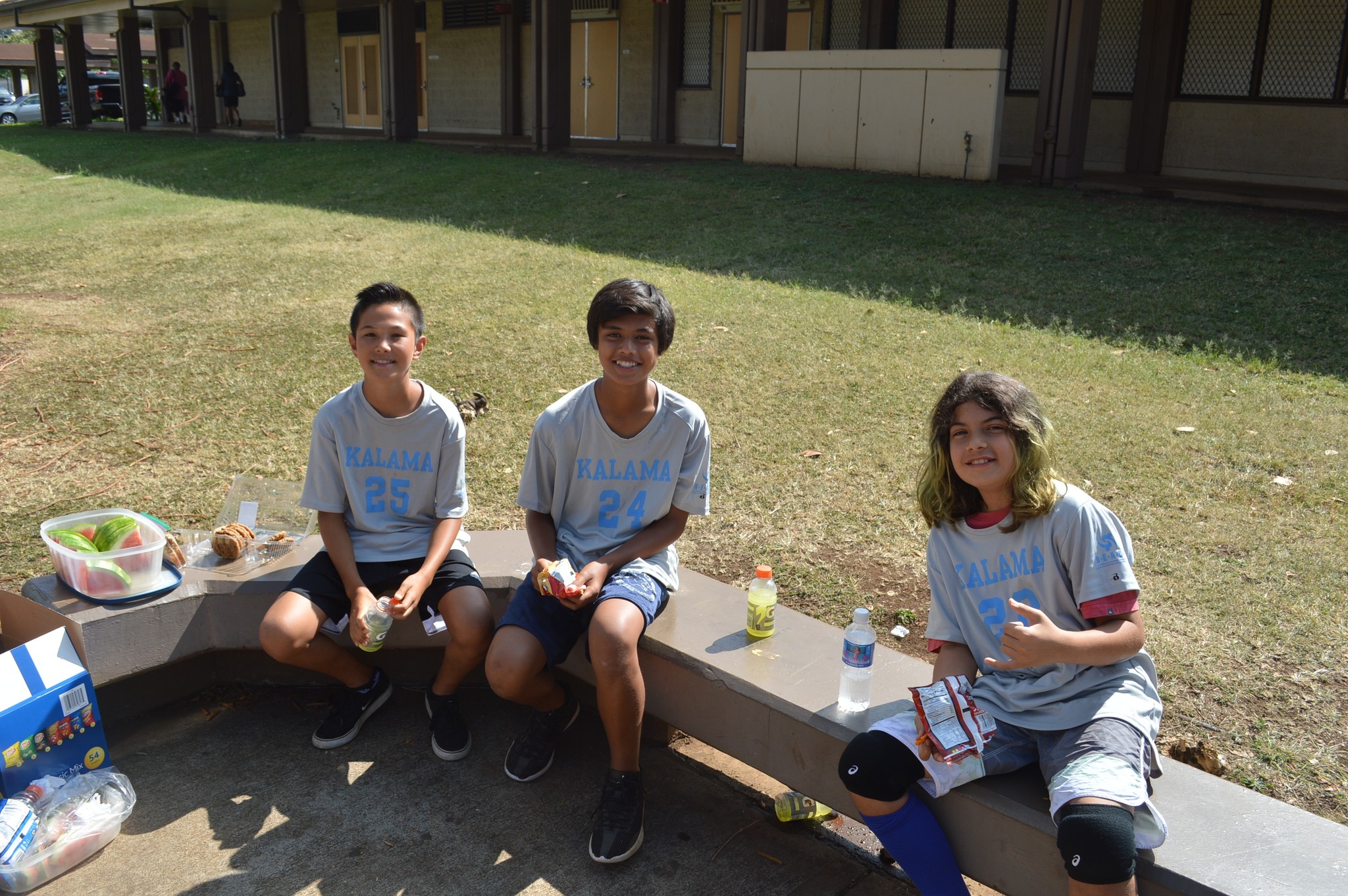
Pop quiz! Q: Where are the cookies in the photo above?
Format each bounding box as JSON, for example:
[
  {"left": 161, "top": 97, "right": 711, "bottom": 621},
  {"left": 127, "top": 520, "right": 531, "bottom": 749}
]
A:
[
  {"left": 258, "top": 530, "right": 296, "bottom": 554},
  {"left": 210, "top": 524, "right": 255, "bottom": 559}
]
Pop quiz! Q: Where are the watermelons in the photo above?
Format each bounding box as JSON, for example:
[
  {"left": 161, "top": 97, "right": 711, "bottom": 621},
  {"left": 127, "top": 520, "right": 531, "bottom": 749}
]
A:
[{"left": 45, "top": 514, "right": 150, "bottom": 598}]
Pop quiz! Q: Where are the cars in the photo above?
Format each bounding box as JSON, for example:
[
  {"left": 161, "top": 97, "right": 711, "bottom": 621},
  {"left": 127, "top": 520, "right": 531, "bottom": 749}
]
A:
[
  {"left": 0, "top": 93, "right": 43, "bottom": 124},
  {"left": 0, "top": 86, "right": 16, "bottom": 106}
]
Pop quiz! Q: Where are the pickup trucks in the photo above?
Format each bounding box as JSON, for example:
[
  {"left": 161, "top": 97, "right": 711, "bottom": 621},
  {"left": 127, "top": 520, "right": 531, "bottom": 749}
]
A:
[{"left": 58, "top": 71, "right": 151, "bottom": 123}]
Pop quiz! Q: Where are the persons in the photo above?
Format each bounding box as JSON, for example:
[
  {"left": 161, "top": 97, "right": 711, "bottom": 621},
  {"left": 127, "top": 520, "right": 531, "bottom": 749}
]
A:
[
  {"left": 163, "top": 61, "right": 187, "bottom": 125},
  {"left": 218, "top": 62, "right": 244, "bottom": 127},
  {"left": 839, "top": 370, "right": 1168, "bottom": 896},
  {"left": 257, "top": 282, "right": 486, "bottom": 762},
  {"left": 485, "top": 277, "right": 711, "bottom": 863}
]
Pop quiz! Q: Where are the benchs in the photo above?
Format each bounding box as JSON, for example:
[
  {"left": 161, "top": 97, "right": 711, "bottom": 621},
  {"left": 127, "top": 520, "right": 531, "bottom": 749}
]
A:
[{"left": 0, "top": 520, "right": 1348, "bottom": 896}]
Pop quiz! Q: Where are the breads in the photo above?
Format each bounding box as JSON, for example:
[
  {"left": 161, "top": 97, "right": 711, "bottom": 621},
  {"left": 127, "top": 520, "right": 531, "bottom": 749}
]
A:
[{"left": 164, "top": 533, "right": 186, "bottom": 568}]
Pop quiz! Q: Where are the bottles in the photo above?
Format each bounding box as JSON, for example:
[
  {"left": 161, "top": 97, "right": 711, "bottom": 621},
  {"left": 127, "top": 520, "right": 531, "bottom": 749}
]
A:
[
  {"left": 775, "top": 791, "right": 832, "bottom": 821},
  {"left": 359, "top": 596, "right": 395, "bottom": 652},
  {"left": 747, "top": 565, "right": 777, "bottom": 638},
  {"left": 10, "top": 785, "right": 43, "bottom": 816},
  {"left": 838, "top": 608, "right": 877, "bottom": 712}
]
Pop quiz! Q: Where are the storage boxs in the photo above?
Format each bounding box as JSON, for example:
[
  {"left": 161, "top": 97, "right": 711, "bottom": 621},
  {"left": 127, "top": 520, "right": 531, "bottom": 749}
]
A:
[
  {"left": 0, "top": 769, "right": 124, "bottom": 894},
  {"left": 0, "top": 625, "right": 116, "bottom": 805},
  {"left": 37, "top": 503, "right": 184, "bottom": 606}
]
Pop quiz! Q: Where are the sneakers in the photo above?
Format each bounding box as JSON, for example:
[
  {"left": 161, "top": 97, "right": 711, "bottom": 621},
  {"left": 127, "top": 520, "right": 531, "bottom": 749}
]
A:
[
  {"left": 504, "top": 691, "right": 579, "bottom": 781},
  {"left": 588, "top": 769, "right": 646, "bottom": 862},
  {"left": 424, "top": 687, "right": 473, "bottom": 761},
  {"left": 312, "top": 668, "right": 392, "bottom": 749}
]
